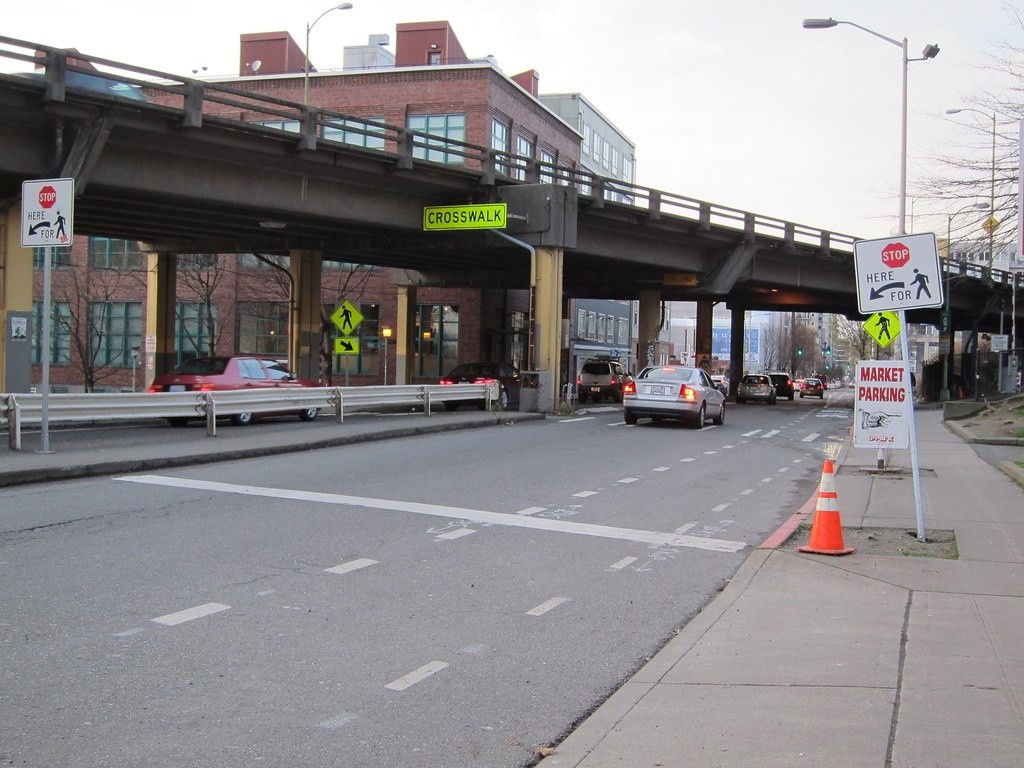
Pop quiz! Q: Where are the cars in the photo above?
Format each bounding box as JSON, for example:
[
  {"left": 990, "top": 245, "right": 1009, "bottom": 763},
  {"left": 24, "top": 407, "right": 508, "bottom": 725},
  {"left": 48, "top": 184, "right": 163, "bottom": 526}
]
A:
[
  {"left": 623, "top": 365, "right": 725, "bottom": 428},
  {"left": 149, "top": 356, "right": 324, "bottom": 425},
  {"left": 440, "top": 362, "right": 522, "bottom": 407},
  {"left": 709, "top": 373, "right": 731, "bottom": 399},
  {"left": 736, "top": 374, "right": 778, "bottom": 404},
  {"left": 792, "top": 378, "right": 805, "bottom": 391},
  {"left": 800, "top": 378, "right": 824, "bottom": 399}
]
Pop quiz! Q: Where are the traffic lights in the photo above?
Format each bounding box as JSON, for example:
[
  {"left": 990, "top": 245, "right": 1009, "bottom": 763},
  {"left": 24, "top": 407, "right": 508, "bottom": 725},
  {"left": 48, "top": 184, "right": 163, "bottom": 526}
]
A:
[
  {"left": 796, "top": 347, "right": 804, "bottom": 355},
  {"left": 825, "top": 364, "right": 830, "bottom": 369},
  {"left": 821, "top": 346, "right": 831, "bottom": 356}
]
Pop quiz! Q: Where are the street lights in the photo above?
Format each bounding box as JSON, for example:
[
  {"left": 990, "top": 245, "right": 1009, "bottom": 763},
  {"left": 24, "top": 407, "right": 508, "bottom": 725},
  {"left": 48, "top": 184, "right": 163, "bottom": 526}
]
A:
[
  {"left": 304, "top": 3, "right": 353, "bottom": 107},
  {"left": 946, "top": 108, "right": 996, "bottom": 279},
  {"left": 910, "top": 190, "right": 944, "bottom": 235},
  {"left": 937, "top": 203, "right": 991, "bottom": 401},
  {"left": 802, "top": 16, "right": 940, "bottom": 236}
]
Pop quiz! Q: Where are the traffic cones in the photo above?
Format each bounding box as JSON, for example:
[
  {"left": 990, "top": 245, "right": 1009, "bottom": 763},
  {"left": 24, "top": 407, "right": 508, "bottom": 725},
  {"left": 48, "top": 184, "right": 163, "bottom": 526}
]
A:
[{"left": 796, "top": 460, "right": 855, "bottom": 554}]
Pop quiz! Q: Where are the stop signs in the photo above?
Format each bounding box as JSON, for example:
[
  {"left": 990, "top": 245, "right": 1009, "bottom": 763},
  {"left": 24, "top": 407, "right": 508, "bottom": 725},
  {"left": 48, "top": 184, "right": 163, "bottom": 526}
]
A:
[
  {"left": 38, "top": 185, "right": 58, "bottom": 208},
  {"left": 881, "top": 242, "right": 911, "bottom": 269}
]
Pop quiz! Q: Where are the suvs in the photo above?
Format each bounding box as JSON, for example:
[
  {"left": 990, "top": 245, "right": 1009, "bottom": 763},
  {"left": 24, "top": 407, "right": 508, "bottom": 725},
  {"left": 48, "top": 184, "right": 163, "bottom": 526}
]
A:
[
  {"left": 769, "top": 373, "right": 796, "bottom": 400},
  {"left": 578, "top": 359, "right": 632, "bottom": 403}
]
